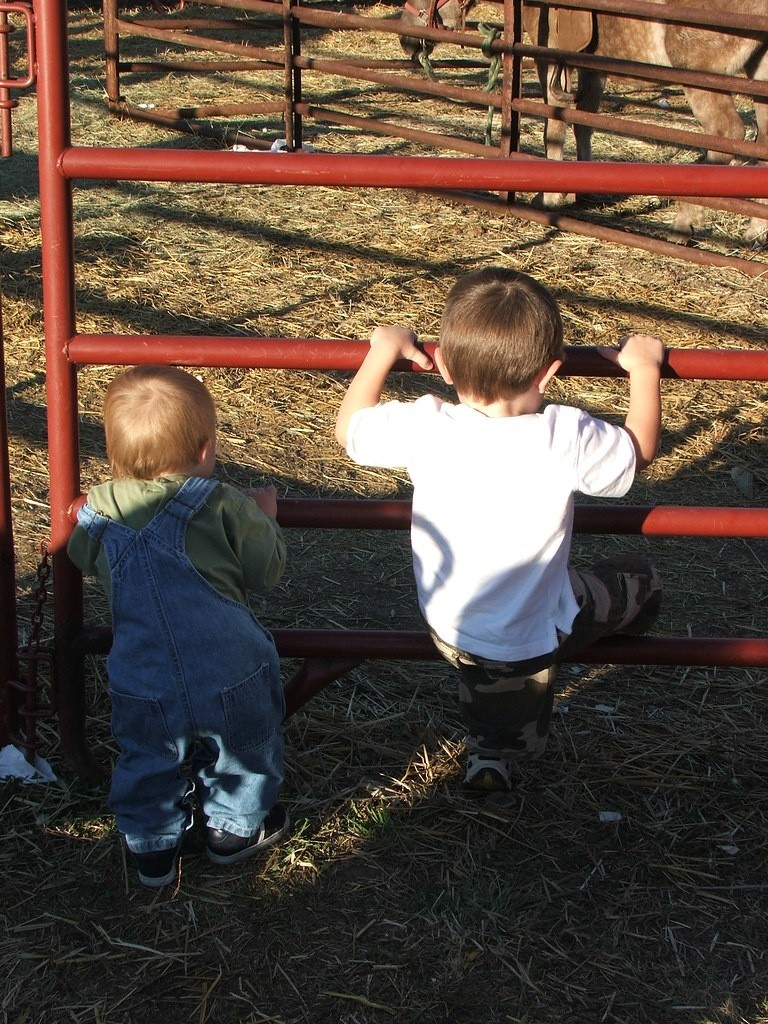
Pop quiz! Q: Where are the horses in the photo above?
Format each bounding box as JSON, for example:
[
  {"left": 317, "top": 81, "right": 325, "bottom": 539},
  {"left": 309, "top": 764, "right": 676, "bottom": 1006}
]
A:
[{"left": 398, "top": 0, "right": 768, "bottom": 247}]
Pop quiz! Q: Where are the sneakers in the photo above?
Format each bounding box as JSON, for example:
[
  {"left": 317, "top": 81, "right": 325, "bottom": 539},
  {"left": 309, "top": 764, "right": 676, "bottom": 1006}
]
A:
[
  {"left": 206, "top": 805, "right": 289, "bottom": 863},
  {"left": 136, "top": 845, "right": 181, "bottom": 887}
]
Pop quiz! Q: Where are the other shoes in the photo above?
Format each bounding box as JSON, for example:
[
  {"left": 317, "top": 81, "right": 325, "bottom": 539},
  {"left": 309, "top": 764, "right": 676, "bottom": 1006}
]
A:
[{"left": 463, "top": 756, "right": 514, "bottom": 793}]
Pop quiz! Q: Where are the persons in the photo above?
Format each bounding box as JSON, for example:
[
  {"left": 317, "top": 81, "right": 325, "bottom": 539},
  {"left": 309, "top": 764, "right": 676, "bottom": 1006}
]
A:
[
  {"left": 336, "top": 269, "right": 663, "bottom": 793},
  {"left": 66, "top": 365, "right": 290, "bottom": 888}
]
